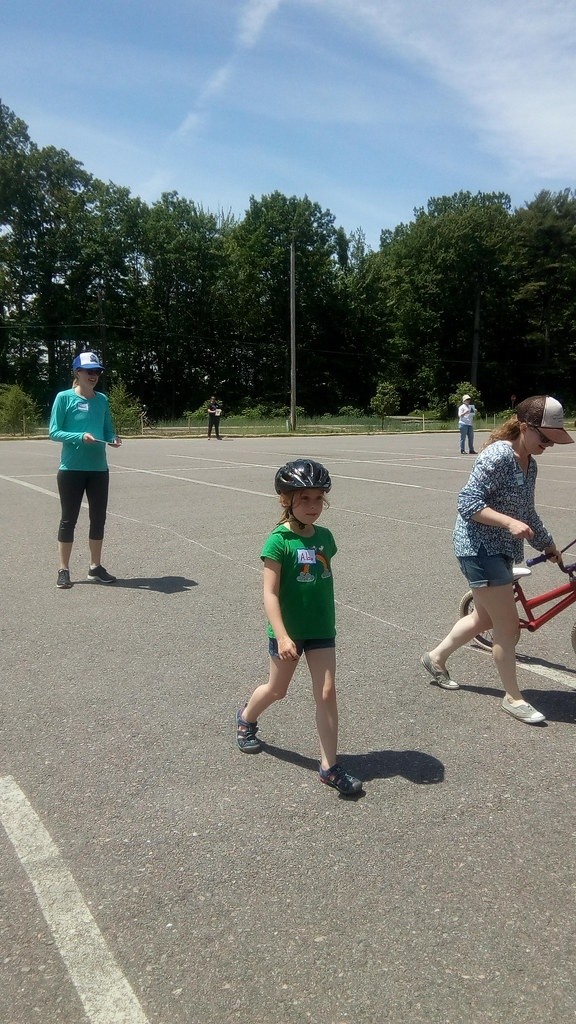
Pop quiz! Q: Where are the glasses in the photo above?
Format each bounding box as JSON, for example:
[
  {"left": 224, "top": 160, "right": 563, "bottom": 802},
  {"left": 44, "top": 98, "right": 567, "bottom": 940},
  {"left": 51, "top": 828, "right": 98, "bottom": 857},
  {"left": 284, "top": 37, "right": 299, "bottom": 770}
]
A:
[
  {"left": 78, "top": 368, "right": 103, "bottom": 376},
  {"left": 535, "top": 426, "right": 549, "bottom": 443}
]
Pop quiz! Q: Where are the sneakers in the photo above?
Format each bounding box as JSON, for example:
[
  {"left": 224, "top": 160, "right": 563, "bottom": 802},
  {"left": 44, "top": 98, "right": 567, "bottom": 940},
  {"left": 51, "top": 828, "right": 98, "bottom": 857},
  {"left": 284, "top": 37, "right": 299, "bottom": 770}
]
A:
[
  {"left": 501, "top": 697, "right": 545, "bottom": 723},
  {"left": 87, "top": 564, "right": 116, "bottom": 582},
  {"left": 56, "top": 569, "right": 71, "bottom": 588},
  {"left": 421, "top": 652, "right": 460, "bottom": 690}
]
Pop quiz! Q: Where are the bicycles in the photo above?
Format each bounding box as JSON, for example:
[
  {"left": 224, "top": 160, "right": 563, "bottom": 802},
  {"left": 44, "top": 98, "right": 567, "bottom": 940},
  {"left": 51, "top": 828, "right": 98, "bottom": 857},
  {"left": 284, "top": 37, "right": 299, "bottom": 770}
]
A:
[{"left": 458, "top": 539, "right": 576, "bottom": 655}]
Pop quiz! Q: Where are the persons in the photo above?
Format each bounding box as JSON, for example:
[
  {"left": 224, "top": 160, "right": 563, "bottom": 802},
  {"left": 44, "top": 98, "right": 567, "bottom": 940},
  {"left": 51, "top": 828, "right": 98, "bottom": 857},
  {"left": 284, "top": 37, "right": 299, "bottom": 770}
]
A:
[
  {"left": 235, "top": 458, "right": 363, "bottom": 795},
  {"left": 207, "top": 396, "right": 222, "bottom": 440},
  {"left": 456, "top": 395, "right": 478, "bottom": 455},
  {"left": 48, "top": 351, "right": 122, "bottom": 588},
  {"left": 418, "top": 395, "right": 575, "bottom": 724}
]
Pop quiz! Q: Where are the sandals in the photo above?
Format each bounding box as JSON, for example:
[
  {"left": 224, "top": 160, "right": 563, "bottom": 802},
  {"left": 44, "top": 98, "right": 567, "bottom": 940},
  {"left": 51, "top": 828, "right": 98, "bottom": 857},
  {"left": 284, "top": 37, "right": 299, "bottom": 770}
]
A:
[
  {"left": 235, "top": 703, "right": 261, "bottom": 754},
  {"left": 317, "top": 758, "right": 362, "bottom": 793}
]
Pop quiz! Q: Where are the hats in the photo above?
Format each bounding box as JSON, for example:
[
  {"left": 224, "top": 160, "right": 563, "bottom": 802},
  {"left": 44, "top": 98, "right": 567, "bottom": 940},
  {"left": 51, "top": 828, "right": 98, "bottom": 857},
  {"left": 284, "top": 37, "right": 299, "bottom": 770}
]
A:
[
  {"left": 462, "top": 394, "right": 471, "bottom": 403},
  {"left": 517, "top": 395, "right": 575, "bottom": 444},
  {"left": 72, "top": 352, "right": 105, "bottom": 372}
]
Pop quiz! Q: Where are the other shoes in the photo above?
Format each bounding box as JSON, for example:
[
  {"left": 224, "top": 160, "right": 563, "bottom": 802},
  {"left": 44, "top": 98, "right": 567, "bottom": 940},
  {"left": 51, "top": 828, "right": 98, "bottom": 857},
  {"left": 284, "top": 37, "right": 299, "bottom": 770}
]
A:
[
  {"left": 218, "top": 437, "right": 222, "bottom": 440},
  {"left": 208, "top": 437, "right": 210, "bottom": 440},
  {"left": 461, "top": 450, "right": 467, "bottom": 454},
  {"left": 469, "top": 450, "right": 478, "bottom": 454}
]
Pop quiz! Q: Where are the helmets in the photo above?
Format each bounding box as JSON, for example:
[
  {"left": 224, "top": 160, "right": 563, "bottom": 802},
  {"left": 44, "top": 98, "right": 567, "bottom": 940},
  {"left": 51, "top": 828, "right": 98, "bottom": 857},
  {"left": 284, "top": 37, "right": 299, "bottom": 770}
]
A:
[{"left": 274, "top": 458, "right": 331, "bottom": 495}]
